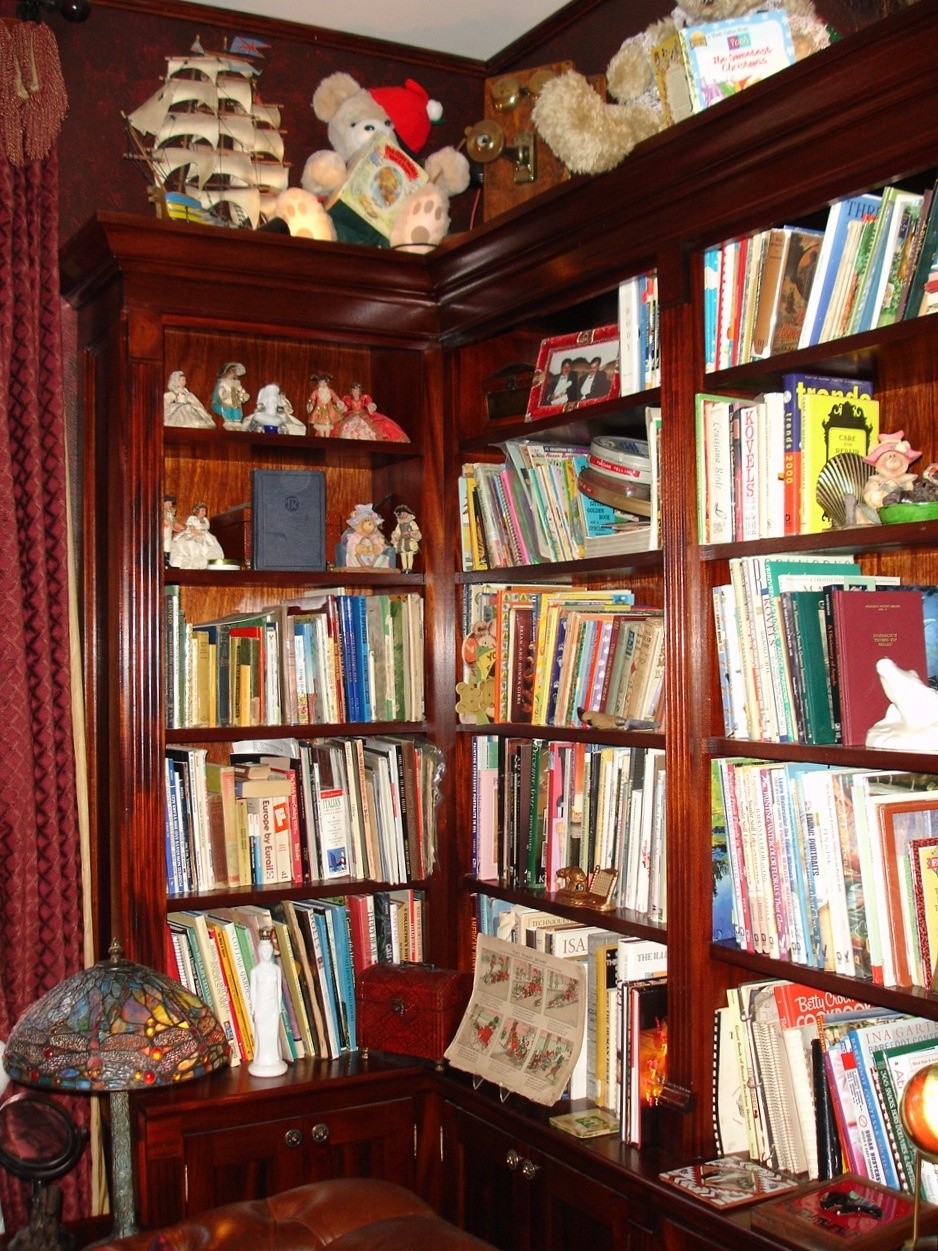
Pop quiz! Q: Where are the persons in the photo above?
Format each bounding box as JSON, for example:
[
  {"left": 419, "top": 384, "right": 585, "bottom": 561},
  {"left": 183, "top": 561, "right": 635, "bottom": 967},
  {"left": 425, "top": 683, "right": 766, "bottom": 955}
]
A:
[
  {"left": 306, "top": 369, "right": 345, "bottom": 437},
  {"left": 212, "top": 362, "right": 251, "bottom": 432},
  {"left": 389, "top": 504, "right": 423, "bottom": 574},
  {"left": 543, "top": 356, "right": 608, "bottom": 406},
  {"left": 330, "top": 381, "right": 411, "bottom": 444},
  {"left": 164, "top": 368, "right": 215, "bottom": 428},
  {"left": 241, "top": 381, "right": 308, "bottom": 437},
  {"left": 247, "top": 927, "right": 288, "bottom": 1078},
  {"left": 162, "top": 493, "right": 224, "bottom": 568}
]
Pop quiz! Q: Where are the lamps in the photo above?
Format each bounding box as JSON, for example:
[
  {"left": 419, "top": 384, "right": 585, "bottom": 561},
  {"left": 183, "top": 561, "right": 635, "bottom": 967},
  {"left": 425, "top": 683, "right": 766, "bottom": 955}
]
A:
[{"left": 4, "top": 938, "right": 231, "bottom": 1239}]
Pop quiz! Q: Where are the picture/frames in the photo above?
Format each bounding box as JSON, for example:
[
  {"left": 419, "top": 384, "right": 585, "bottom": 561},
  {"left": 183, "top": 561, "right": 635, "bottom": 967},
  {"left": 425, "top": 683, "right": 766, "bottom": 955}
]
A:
[{"left": 525, "top": 323, "right": 620, "bottom": 423}]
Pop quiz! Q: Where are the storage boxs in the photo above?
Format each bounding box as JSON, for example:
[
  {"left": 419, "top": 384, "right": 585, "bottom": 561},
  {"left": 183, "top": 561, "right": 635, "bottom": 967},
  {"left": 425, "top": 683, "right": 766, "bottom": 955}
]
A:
[{"left": 751, "top": 1173, "right": 938, "bottom": 1251}]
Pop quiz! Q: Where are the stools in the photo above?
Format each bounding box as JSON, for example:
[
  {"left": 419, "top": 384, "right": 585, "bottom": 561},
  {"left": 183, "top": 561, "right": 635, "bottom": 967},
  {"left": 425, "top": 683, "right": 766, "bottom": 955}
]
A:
[{"left": 84, "top": 1176, "right": 503, "bottom": 1251}]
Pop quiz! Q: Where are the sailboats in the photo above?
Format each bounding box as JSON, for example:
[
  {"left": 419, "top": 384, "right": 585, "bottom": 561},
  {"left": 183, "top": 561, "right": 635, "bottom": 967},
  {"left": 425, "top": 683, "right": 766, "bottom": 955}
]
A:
[{"left": 118, "top": 34, "right": 292, "bottom": 232}]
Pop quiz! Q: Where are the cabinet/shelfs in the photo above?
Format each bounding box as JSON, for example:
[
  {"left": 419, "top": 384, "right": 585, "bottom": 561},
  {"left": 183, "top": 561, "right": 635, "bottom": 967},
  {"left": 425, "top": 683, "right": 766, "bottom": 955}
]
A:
[{"left": 58, "top": 0, "right": 938, "bottom": 1251}]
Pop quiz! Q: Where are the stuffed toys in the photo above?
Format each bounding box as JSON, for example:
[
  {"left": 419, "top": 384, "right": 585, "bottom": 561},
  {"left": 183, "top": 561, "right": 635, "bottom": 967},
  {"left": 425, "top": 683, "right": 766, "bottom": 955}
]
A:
[
  {"left": 273, "top": 72, "right": 472, "bottom": 255},
  {"left": 530, "top": 0, "right": 831, "bottom": 176}
]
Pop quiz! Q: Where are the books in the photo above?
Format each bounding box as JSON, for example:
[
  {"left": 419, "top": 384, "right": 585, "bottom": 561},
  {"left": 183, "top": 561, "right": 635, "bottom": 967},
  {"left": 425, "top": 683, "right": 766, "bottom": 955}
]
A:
[
  {"left": 469, "top": 890, "right": 674, "bottom": 1151},
  {"left": 713, "top": 557, "right": 928, "bottom": 746},
  {"left": 694, "top": 373, "right": 879, "bottom": 544},
  {"left": 712, "top": 980, "right": 938, "bottom": 1203},
  {"left": 460, "top": 406, "right": 664, "bottom": 573},
  {"left": 616, "top": 266, "right": 663, "bottom": 398},
  {"left": 704, "top": 180, "right": 938, "bottom": 373},
  {"left": 164, "top": 735, "right": 443, "bottom": 895},
  {"left": 250, "top": 468, "right": 328, "bottom": 572},
  {"left": 460, "top": 582, "right": 667, "bottom": 730},
  {"left": 470, "top": 734, "right": 669, "bottom": 924},
  {"left": 710, "top": 758, "right": 938, "bottom": 990},
  {"left": 163, "top": 583, "right": 427, "bottom": 727},
  {"left": 165, "top": 887, "right": 425, "bottom": 1068}
]
